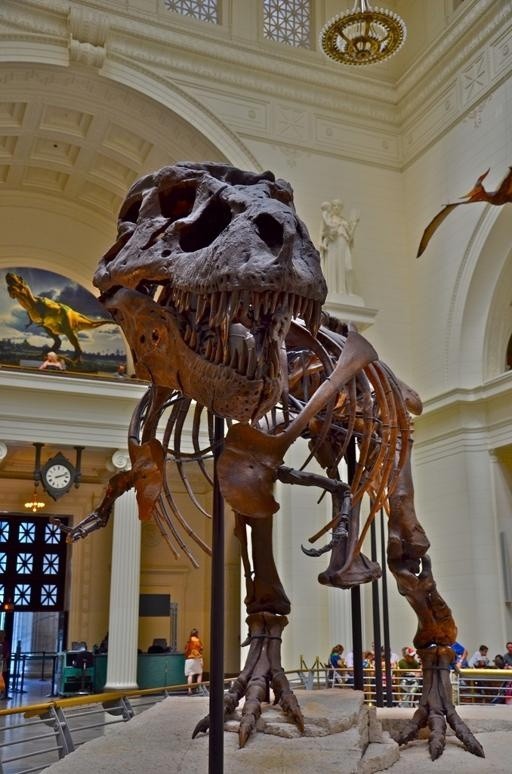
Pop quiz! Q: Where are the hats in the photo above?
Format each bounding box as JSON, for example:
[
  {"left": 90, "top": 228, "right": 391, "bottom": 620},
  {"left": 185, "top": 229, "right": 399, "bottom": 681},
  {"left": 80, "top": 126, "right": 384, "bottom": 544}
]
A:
[{"left": 402, "top": 647, "right": 416, "bottom": 656}]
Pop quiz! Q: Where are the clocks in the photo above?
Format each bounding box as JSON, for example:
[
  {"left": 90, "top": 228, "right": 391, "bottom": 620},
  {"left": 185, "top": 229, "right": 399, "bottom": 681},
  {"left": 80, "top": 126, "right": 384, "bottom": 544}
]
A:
[{"left": 32, "top": 442, "right": 86, "bottom": 502}]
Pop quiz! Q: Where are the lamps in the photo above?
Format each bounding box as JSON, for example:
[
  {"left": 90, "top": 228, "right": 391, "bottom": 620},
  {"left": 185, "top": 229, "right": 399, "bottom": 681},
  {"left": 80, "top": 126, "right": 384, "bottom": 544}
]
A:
[
  {"left": 318, "top": 0, "right": 408, "bottom": 68},
  {"left": 24, "top": 487, "right": 45, "bottom": 513}
]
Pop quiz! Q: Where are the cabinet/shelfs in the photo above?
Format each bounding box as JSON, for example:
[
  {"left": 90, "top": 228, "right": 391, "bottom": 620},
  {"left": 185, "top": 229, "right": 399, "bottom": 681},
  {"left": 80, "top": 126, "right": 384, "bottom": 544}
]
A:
[{"left": 59, "top": 651, "right": 95, "bottom": 697}]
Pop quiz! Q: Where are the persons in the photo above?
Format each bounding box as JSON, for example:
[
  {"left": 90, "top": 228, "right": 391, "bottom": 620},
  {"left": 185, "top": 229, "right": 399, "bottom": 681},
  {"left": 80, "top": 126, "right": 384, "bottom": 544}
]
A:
[
  {"left": 113, "top": 365, "right": 129, "bottom": 378},
  {"left": 318, "top": 198, "right": 361, "bottom": 297},
  {"left": 40, "top": 352, "right": 66, "bottom": 369},
  {"left": 328, "top": 644, "right": 420, "bottom": 706},
  {"left": 184, "top": 628, "right": 205, "bottom": 694},
  {"left": 448, "top": 641, "right": 512, "bottom": 704}
]
